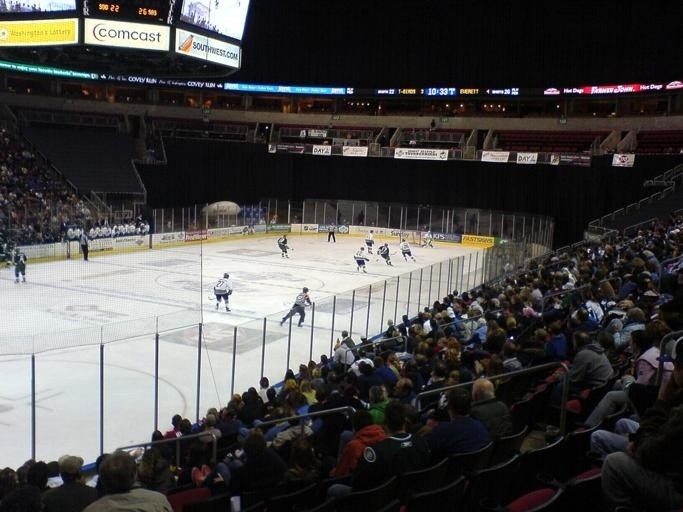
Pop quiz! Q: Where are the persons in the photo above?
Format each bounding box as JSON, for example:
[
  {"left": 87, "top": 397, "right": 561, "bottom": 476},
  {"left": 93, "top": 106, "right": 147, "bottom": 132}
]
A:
[
  {"left": 0, "top": 287, "right": 683, "bottom": 512},
  {"left": 480, "top": 214, "right": 682, "bottom": 288},
  {"left": 214, "top": 273, "right": 232, "bottom": 311},
  {"left": 377, "top": 244, "right": 393, "bottom": 267},
  {"left": 365, "top": 229, "right": 374, "bottom": 254},
  {"left": 1, "top": 101, "right": 149, "bottom": 264},
  {"left": 353, "top": 248, "right": 369, "bottom": 273},
  {"left": 276, "top": 233, "right": 293, "bottom": 259},
  {"left": 399, "top": 239, "right": 415, "bottom": 262},
  {"left": 164, "top": 212, "right": 429, "bottom": 235},
  {"left": 428, "top": 238, "right": 433, "bottom": 249},
  {"left": 326, "top": 118, "right": 436, "bottom": 147},
  {"left": 180, "top": 0, "right": 241, "bottom": 35},
  {"left": 0, "top": 0, "right": 49, "bottom": 13},
  {"left": 13, "top": 247, "right": 26, "bottom": 282},
  {"left": 327, "top": 222, "right": 337, "bottom": 243},
  {"left": 422, "top": 237, "right": 427, "bottom": 248}
]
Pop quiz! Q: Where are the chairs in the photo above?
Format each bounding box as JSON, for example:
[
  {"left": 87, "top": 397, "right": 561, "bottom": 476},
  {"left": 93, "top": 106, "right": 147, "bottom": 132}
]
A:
[{"left": 169, "top": 210, "right": 682, "bottom": 511}]
